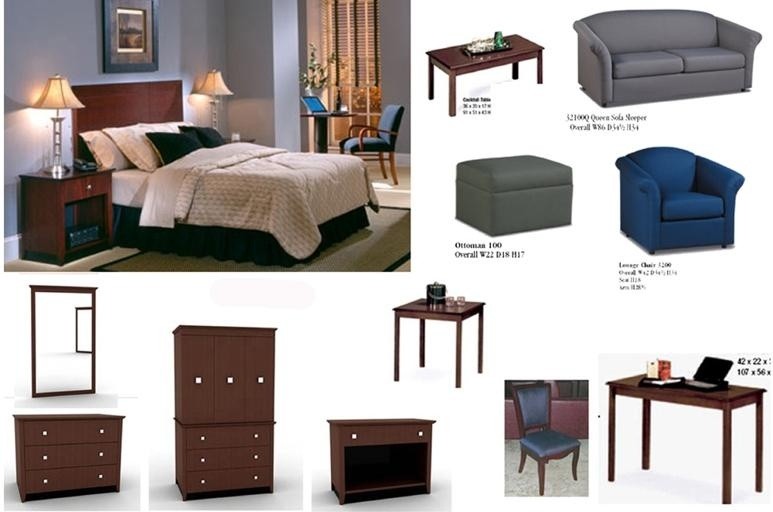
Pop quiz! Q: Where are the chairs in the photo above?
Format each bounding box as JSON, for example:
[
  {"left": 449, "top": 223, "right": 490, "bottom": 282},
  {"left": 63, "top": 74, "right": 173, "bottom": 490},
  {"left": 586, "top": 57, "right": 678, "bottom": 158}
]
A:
[
  {"left": 508, "top": 382, "right": 581, "bottom": 497},
  {"left": 338, "top": 104, "right": 404, "bottom": 186}
]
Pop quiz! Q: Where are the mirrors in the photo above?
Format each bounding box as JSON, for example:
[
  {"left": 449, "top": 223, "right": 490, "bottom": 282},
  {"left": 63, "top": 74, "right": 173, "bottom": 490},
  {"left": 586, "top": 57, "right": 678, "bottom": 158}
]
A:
[
  {"left": 28, "top": 284, "right": 98, "bottom": 399},
  {"left": 74, "top": 306, "right": 92, "bottom": 353}
]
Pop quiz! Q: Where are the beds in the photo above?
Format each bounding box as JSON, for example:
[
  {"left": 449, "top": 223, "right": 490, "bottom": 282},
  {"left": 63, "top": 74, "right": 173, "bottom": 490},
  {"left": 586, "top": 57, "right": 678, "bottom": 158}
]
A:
[{"left": 71, "top": 80, "right": 380, "bottom": 268}]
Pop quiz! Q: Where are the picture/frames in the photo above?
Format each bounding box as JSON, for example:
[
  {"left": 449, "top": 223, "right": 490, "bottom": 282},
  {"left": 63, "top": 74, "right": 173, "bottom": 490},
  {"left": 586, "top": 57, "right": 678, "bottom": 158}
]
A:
[{"left": 102, "top": 0, "right": 159, "bottom": 74}]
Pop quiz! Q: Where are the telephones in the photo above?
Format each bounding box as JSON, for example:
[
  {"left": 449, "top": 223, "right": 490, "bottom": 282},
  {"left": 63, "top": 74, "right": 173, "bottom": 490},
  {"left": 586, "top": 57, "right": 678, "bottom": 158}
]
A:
[{"left": 72, "top": 158, "right": 98, "bottom": 173}]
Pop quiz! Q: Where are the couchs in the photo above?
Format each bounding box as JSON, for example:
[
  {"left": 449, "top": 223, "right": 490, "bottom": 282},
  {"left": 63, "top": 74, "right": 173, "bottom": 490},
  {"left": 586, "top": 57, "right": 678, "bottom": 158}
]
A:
[
  {"left": 455, "top": 156, "right": 573, "bottom": 238},
  {"left": 571, "top": 8, "right": 761, "bottom": 108},
  {"left": 615, "top": 147, "right": 746, "bottom": 255}
]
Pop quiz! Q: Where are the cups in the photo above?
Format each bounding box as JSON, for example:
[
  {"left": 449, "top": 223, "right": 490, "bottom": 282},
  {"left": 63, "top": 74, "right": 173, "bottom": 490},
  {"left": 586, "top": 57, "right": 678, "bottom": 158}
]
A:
[
  {"left": 456, "top": 295, "right": 465, "bottom": 307},
  {"left": 340, "top": 103, "right": 348, "bottom": 112},
  {"left": 230, "top": 130, "right": 240, "bottom": 142},
  {"left": 445, "top": 297, "right": 453, "bottom": 307}
]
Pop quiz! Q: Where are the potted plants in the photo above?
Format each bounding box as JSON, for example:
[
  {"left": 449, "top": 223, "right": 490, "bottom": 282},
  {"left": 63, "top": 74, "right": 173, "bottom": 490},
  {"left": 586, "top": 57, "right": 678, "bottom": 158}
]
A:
[{"left": 299, "top": 43, "right": 350, "bottom": 115}]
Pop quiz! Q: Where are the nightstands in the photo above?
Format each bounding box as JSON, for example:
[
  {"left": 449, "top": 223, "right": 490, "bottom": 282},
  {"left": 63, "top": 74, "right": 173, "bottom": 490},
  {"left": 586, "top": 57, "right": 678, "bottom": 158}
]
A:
[
  {"left": 218, "top": 136, "right": 256, "bottom": 144},
  {"left": 17, "top": 167, "right": 116, "bottom": 267}
]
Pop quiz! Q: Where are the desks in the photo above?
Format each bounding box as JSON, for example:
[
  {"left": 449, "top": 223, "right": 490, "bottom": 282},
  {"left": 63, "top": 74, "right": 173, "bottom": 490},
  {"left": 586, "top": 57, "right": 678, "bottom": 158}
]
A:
[
  {"left": 300, "top": 111, "right": 357, "bottom": 153},
  {"left": 391, "top": 297, "right": 486, "bottom": 388},
  {"left": 604, "top": 373, "right": 767, "bottom": 503}
]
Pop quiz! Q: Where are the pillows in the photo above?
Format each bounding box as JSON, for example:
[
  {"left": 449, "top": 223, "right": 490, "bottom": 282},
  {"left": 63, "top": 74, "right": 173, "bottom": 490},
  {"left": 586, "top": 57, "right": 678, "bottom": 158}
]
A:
[
  {"left": 144, "top": 131, "right": 204, "bottom": 167},
  {"left": 177, "top": 126, "right": 225, "bottom": 149},
  {"left": 162, "top": 122, "right": 196, "bottom": 134},
  {"left": 100, "top": 124, "right": 176, "bottom": 174},
  {"left": 76, "top": 130, "right": 136, "bottom": 171}
]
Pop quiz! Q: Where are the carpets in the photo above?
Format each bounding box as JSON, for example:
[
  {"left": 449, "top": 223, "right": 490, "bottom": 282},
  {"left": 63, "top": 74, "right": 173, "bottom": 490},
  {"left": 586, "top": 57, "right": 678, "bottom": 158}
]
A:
[{"left": 89, "top": 202, "right": 409, "bottom": 271}]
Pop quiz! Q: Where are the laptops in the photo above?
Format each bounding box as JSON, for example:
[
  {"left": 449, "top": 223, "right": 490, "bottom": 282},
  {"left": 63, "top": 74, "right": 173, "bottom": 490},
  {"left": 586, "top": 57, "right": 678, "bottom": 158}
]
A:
[{"left": 300, "top": 96, "right": 348, "bottom": 115}]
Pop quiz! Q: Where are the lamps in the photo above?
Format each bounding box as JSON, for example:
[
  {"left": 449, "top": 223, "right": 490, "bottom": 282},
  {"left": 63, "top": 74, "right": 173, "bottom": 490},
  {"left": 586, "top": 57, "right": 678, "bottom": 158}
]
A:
[
  {"left": 31, "top": 73, "right": 86, "bottom": 173},
  {"left": 190, "top": 69, "right": 234, "bottom": 131}
]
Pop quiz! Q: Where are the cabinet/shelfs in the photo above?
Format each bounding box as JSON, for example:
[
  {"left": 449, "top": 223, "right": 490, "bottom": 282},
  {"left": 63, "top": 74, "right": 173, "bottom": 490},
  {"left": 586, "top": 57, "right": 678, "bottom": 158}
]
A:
[
  {"left": 172, "top": 324, "right": 276, "bottom": 424},
  {"left": 327, "top": 418, "right": 436, "bottom": 506},
  {"left": 12, "top": 412, "right": 126, "bottom": 503},
  {"left": 173, "top": 420, "right": 276, "bottom": 503}
]
